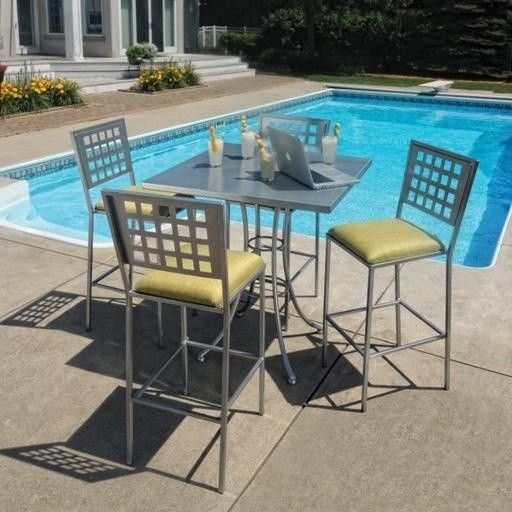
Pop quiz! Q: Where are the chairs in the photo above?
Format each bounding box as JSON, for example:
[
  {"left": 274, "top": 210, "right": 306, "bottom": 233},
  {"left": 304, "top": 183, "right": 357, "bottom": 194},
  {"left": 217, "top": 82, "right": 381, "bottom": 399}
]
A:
[
  {"left": 319, "top": 137, "right": 478, "bottom": 409},
  {"left": 231, "top": 115, "right": 345, "bottom": 328},
  {"left": 71, "top": 115, "right": 200, "bottom": 347},
  {"left": 98, "top": 186, "right": 268, "bottom": 494}
]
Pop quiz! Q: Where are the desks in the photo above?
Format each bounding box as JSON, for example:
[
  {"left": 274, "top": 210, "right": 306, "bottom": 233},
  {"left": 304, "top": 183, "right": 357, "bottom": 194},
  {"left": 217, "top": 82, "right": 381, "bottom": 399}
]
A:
[{"left": 139, "top": 141, "right": 375, "bottom": 387}]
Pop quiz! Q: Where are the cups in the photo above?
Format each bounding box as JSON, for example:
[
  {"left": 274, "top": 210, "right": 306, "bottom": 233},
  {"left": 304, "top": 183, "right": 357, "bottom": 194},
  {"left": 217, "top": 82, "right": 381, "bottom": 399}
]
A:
[
  {"left": 320, "top": 135, "right": 338, "bottom": 165},
  {"left": 240, "top": 132, "right": 256, "bottom": 159},
  {"left": 259, "top": 151, "right": 276, "bottom": 182},
  {"left": 207, "top": 139, "right": 224, "bottom": 165}
]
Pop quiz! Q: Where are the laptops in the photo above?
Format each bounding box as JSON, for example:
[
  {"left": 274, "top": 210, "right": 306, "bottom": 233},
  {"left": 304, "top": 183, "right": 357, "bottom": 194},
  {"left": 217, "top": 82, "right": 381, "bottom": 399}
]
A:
[{"left": 266, "top": 126, "right": 361, "bottom": 191}]
